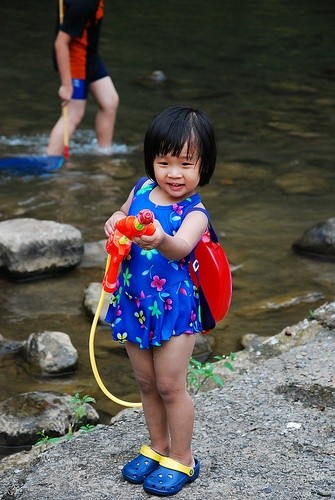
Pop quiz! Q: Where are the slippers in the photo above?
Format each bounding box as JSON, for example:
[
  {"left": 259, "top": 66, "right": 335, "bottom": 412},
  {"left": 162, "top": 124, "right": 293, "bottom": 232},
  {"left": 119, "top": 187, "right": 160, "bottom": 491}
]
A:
[
  {"left": 144, "top": 456, "right": 201, "bottom": 496},
  {"left": 123, "top": 443, "right": 171, "bottom": 483}
]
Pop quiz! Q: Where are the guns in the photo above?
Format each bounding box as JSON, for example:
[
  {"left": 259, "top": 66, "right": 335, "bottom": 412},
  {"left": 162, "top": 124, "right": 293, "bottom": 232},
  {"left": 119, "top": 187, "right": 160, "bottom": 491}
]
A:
[{"left": 96, "top": 207, "right": 156, "bottom": 295}]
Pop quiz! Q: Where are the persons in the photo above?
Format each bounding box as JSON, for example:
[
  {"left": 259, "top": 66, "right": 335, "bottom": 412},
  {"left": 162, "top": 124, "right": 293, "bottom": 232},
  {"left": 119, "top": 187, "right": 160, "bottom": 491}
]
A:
[
  {"left": 105, "top": 105, "right": 219, "bottom": 496},
  {"left": 46, "top": 0, "right": 119, "bottom": 157}
]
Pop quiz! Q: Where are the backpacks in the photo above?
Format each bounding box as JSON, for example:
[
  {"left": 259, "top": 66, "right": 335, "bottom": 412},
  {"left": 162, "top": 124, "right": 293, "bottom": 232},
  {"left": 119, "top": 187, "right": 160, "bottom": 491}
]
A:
[{"left": 134, "top": 176, "right": 232, "bottom": 335}]
